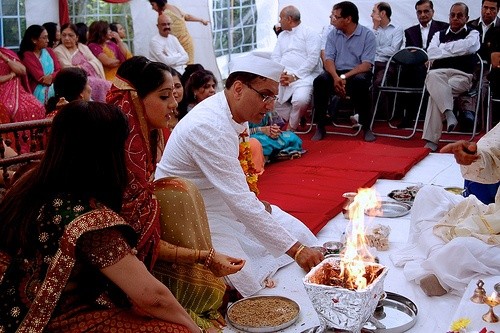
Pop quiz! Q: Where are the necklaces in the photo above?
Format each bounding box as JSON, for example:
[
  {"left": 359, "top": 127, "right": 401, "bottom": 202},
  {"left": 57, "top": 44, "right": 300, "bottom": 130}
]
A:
[{"left": 238, "top": 129, "right": 260, "bottom": 197}]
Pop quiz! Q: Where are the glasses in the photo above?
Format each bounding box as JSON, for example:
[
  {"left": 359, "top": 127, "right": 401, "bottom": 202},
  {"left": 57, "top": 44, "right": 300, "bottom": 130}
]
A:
[
  {"left": 243, "top": 83, "right": 282, "bottom": 107},
  {"left": 330, "top": 13, "right": 345, "bottom": 23}
]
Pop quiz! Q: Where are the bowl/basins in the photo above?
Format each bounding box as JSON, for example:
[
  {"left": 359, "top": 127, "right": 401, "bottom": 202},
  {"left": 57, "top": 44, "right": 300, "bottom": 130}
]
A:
[
  {"left": 323, "top": 241, "right": 343, "bottom": 254},
  {"left": 310, "top": 246, "right": 327, "bottom": 256}
]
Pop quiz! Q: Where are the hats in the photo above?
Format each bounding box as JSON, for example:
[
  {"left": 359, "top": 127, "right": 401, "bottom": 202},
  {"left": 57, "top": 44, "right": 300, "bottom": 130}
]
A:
[{"left": 227, "top": 55, "right": 285, "bottom": 82}]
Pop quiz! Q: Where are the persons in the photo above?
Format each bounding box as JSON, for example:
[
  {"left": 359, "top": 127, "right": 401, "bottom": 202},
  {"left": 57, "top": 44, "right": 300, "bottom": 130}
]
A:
[
  {"left": 266, "top": 3, "right": 321, "bottom": 132},
  {"left": 166, "top": 62, "right": 308, "bottom": 164},
  {"left": 0, "top": 99, "right": 220, "bottom": 333},
  {"left": 420, "top": 2, "right": 481, "bottom": 152},
  {"left": 149, "top": 0, "right": 209, "bottom": 73},
  {"left": 370, "top": 2, "right": 406, "bottom": 119},
  {"left": 389, "top": 121, "right": 500, "bottom": 296},
  {"left": 396, "top": 0, "right": 449, "bottom": 128},
  {"left": 0, "top": 20, "right": 146, "bottom": 154},
  {"left": 310, "top": 2, "right": 376, "bottom": 142},
  {"left": 101, "top": 53, "right": 247, "bottom": 333},
  {"left": 459, "top": 0, "right": 500, "bottom": 122}
]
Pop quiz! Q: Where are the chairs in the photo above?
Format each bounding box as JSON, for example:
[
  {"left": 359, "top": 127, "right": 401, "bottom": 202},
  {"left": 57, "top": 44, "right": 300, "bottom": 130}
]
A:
[{"left": 294, "top": 46, "right": 500, "bottom": 144}]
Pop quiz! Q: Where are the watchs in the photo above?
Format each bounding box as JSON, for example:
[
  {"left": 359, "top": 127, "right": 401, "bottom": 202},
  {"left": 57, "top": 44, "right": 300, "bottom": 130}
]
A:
[{"left": 339, "top": 74, "right": 346, "bottom": 81}]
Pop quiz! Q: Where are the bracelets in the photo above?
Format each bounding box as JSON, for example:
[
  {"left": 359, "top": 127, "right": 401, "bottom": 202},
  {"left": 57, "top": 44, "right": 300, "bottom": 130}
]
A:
[
  {"left": 291, "top": 73, "right": 297, "bottom": 81},
  {"left": 204, "top": 247, "right": 215, "bottom": 271},
  {"left": 191, "top": 246, "right": 202, "bottom": 264},
  {"left": 198, "top": 326, "right": 208, "bottom": 332},
  {"left": 293, "top": 242, "right": 306, "bottom": 262},
  {"left": 174, "top": 244, "right": 179, "bottom": 265}
]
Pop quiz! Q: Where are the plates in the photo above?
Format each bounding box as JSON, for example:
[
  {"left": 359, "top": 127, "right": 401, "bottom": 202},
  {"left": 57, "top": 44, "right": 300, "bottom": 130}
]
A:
[
  {"left": 226, "top": 295, "right": 301, "bottom": 333},
  {"left": 362, "top": 290, "right": 418, "bottom": 333},
  {"left": 443, "top": 187, "right": 464, "bottom": 196},
  {"left": 363, "top": 201, "right": 412, "bottom": 218},
  {"left": 387, "top": 188, "right": 419, "bottom": 204}
]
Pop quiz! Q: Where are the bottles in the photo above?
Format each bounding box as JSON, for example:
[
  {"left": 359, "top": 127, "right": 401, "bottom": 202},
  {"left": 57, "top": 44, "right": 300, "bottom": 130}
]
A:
[{"left": 342, "top": 192, "right": 359, "bottom": 220}]
